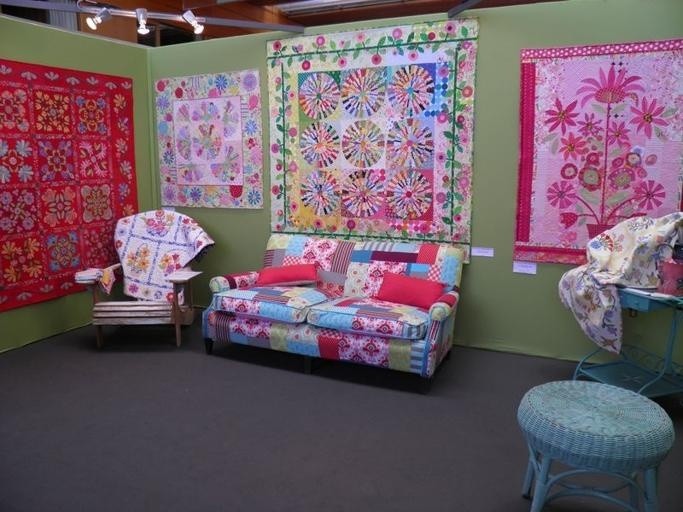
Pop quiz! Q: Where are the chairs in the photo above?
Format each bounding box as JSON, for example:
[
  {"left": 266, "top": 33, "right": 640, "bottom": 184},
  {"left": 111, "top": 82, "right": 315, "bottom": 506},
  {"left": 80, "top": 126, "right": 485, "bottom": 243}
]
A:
[{"left": 74, "top": 210, "right": 204, "bottom": 351}]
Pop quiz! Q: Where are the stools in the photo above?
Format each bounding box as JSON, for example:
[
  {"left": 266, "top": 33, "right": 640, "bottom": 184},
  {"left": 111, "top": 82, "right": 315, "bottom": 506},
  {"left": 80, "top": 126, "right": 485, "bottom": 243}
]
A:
[{"left": 517, "top": 378, "right": 676, "bottom": 510}]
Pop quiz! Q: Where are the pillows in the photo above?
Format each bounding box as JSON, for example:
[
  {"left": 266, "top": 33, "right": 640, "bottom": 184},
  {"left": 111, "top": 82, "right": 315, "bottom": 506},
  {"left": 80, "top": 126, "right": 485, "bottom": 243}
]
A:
[
  {"left": 257, "top": 263, "right": 319, "bottom": 288},
  {"left": 376, "top": 270, "right": 444, "bottom": 310}
]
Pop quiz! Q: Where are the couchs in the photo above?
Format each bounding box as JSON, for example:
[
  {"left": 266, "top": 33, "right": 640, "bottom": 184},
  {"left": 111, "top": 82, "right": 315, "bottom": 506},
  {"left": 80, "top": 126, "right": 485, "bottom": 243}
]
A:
[{"left": 200, "top": 233, "right": 466, "bottom": 394}]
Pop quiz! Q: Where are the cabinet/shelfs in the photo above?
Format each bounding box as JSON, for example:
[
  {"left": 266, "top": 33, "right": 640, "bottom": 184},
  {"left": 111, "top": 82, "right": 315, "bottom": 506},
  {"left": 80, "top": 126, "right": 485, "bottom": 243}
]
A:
[{"left": 572, "top": 285, "right": 682, "bottom": 400}]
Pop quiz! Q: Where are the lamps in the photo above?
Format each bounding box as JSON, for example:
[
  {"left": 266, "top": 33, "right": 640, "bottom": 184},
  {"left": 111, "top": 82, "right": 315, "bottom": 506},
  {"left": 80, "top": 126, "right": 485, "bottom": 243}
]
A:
[
  {"left": 181, "top": 10, "right": 208, "bottom": 34},
  {"left": 82, "top": 6, "right": 112, "bottom": 30},
  {"left": 134, "top": 7, "right": 151, "bottom": 36}
]
